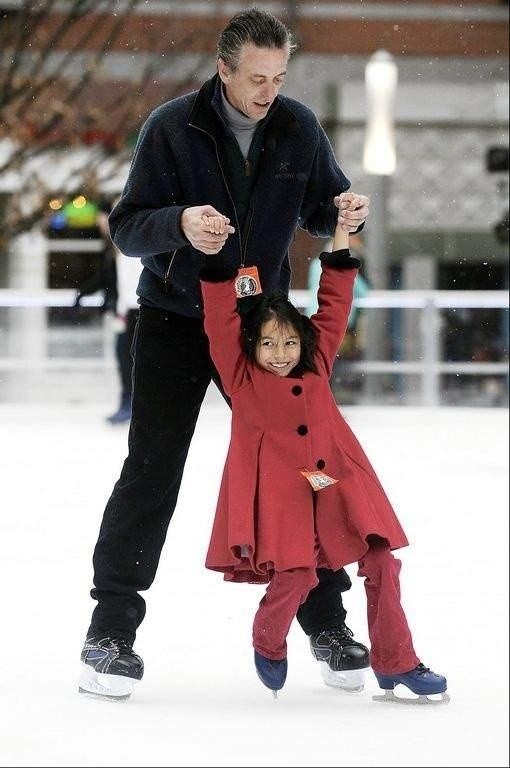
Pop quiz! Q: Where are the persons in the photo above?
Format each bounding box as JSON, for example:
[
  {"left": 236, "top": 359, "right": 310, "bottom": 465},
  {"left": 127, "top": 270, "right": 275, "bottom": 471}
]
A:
[
  {"left": 79, "top": 6, "right": 372, "bottom": 681},
  {"left": 70, "top": 193, "right": 145, "bottom": 425},
  {"left": 199, "top": 191, "right": 448, "bottom": 695}
]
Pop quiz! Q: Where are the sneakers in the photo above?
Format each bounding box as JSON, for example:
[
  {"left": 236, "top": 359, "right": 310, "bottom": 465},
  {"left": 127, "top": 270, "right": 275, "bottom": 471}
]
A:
[
  {"left": 310, "top": 623, "right": 370, "bottom": 671},
  {"left": 373, "top": 663, "right": 448, "bottom": 696},
  {"left": 254, "top": 649, "right": 287, "bottom": 690},
  {"left": 107, "top": 410, "right": 131, "bottom": 424},
  {"left": 81, "top": 632, "right": 145, "bottom": 682}
]
